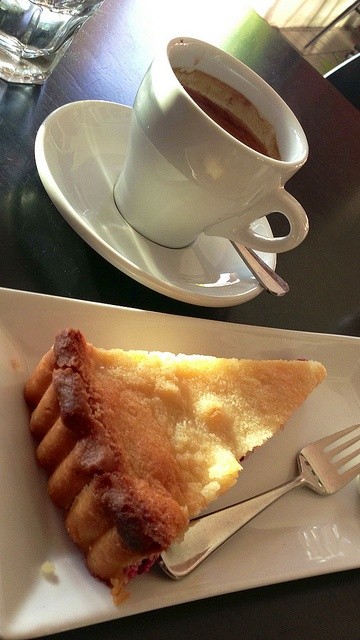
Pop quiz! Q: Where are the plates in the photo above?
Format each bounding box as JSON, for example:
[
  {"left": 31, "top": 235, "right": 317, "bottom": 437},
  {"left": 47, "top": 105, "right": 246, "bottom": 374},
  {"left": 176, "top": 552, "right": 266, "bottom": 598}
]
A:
[
  {"left": 35, "top": 100, "right": 277, "bottom": 307},
  {"left": 0, "top": 287, "right": 360, "bottom": 640}
]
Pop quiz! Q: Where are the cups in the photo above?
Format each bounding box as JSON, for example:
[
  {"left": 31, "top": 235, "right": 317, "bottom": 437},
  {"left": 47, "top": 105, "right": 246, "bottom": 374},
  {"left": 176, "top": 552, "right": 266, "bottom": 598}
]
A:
[
  {"left": 0, "top": 0, "right": 104, "bottom": 84},
  {"left": 114, "top": 37, "right": 310, "bottom": 253}
]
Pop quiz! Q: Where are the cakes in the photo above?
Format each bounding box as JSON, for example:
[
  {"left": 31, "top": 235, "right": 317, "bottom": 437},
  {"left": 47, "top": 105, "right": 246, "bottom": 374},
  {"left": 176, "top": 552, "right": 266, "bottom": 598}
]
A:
[{"left": 23, "top": 329, "right": 328, "bottom": 607}]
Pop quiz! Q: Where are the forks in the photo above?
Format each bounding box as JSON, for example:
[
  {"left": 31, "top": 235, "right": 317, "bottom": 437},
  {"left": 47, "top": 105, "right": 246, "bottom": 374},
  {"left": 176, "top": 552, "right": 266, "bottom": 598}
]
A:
[{"left": 156, "top": 423, "right": 360, "bottom": 580}]
